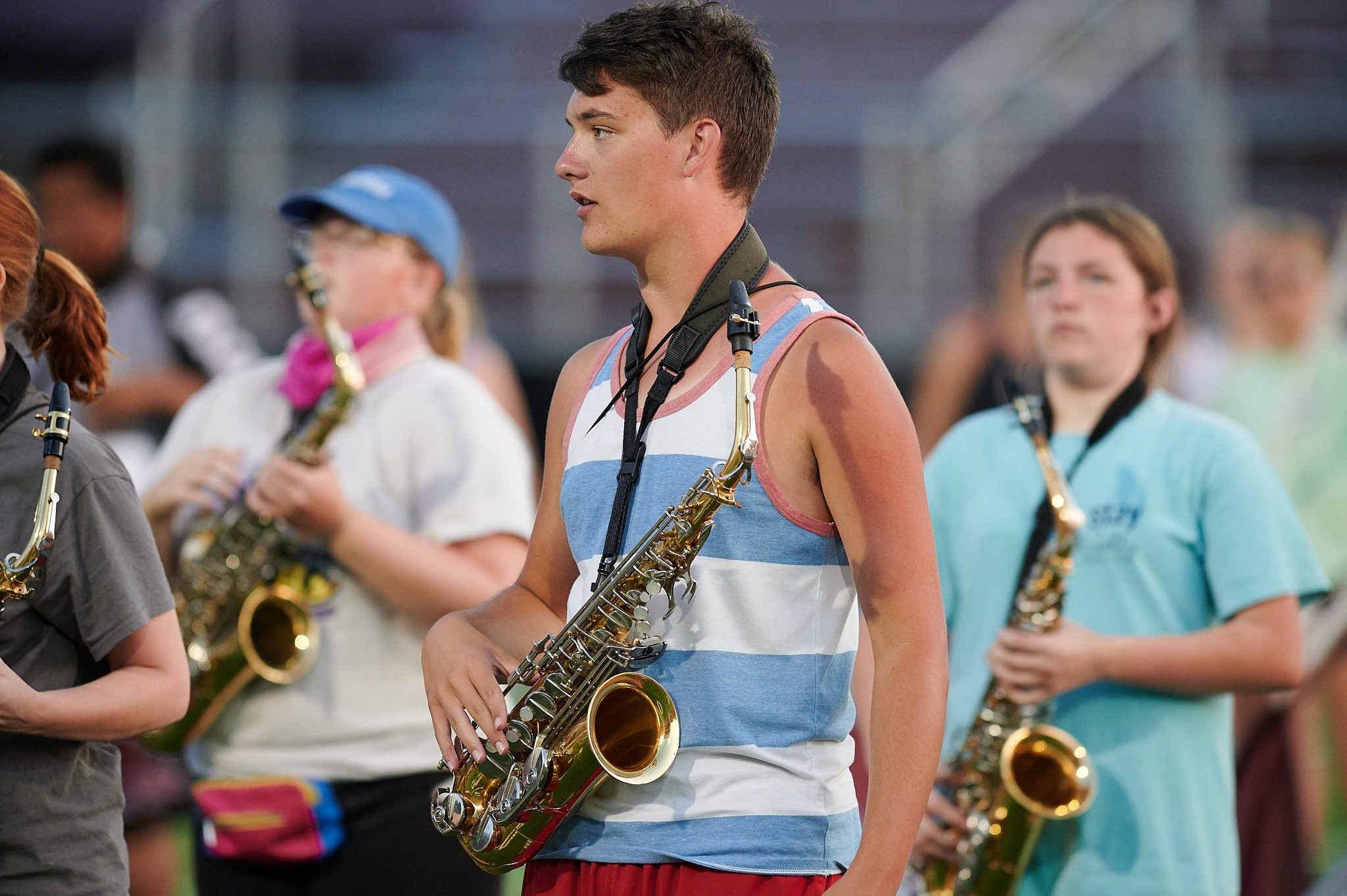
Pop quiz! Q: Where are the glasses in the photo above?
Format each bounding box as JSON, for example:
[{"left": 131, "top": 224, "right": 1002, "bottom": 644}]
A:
[{"left": 290, "top": 224, "right": 391, "bottom": 261}]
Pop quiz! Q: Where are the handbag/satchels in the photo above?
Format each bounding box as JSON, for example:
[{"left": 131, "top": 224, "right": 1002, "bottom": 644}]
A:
[{"left": 186, "top": 778, "right": 353, "bottom": 863}]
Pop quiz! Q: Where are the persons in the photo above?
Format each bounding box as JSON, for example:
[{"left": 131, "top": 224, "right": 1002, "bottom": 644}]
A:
[
  {"left": 144, "top": 170, "right": 536, "bottom": 896},
  {"left": 421, "top": 0, "right": 951, "bottom": 896},
  {"left": 0, "top": 176, "right": 193, "bottom": 896},
  {"left": 30, "top": 135, "right": 206, "bottom": 505},
  {"left": 1168, "top": 215, "right": 1347, "bottom": 896},
  {"left": 853, "top": 189, "right": 1331, "bottom": 896},
  {"left": 910, "top": 194, "right": 1074, "bottom": 453}
]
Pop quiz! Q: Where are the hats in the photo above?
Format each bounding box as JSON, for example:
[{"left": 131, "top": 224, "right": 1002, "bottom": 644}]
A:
[{"left": 276, "top": 163, "right": 461, "bottom": 282}]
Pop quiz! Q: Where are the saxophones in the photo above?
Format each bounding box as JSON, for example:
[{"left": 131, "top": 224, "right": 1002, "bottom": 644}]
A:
[
  {"left": 905, "top": 361, "right": 1098, "bottom": 896},
  {"left": 429, "top": 272, "right": 765, "bottom": 875},
  {"left": 0, "top": 379, "right": 74, "bottom": 614},
  {"left": 131, "top": 239, "right": 367, "bottom": 759}
]
